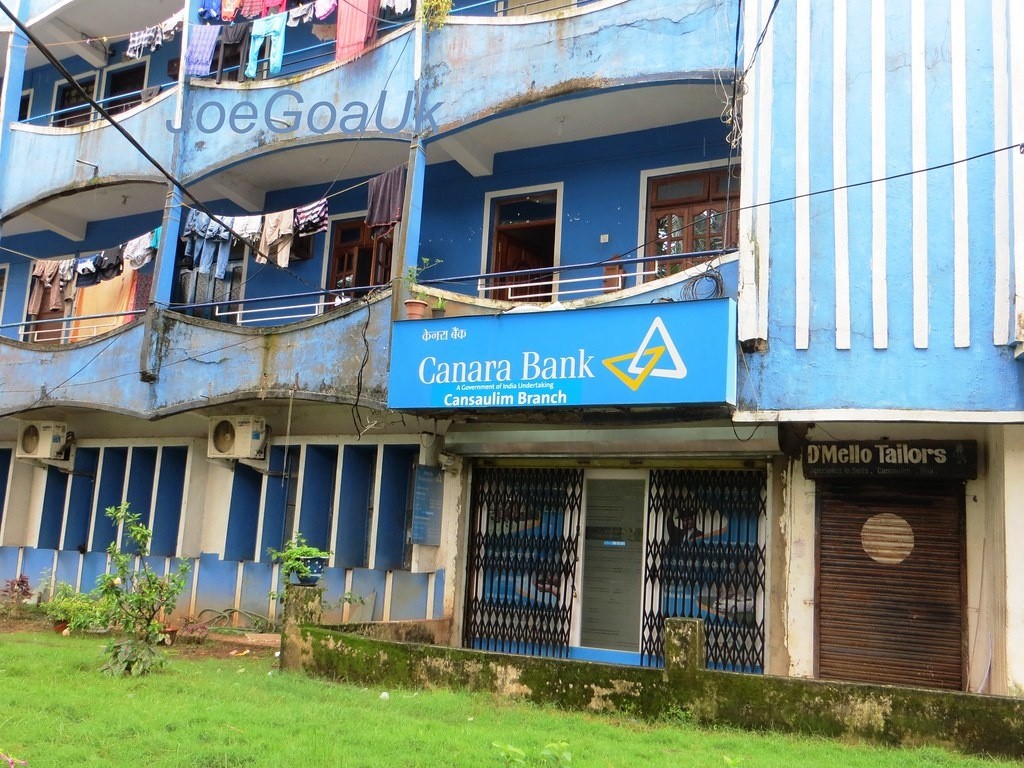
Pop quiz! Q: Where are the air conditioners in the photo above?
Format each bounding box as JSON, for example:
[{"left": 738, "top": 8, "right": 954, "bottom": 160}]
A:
[
  {"left": 252, "top": 222, "right": 313, "bottom": 265},
  {"left": 206, "top": 414, "right": 266, "bottom": 458},
  {"left": 15, "top": 420, "right": 67, "bottom": 460}
]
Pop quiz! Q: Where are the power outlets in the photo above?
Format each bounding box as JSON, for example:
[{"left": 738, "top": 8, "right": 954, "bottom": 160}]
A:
[{"left": 600, "top": 234, "right": 609, "bottom": 244}]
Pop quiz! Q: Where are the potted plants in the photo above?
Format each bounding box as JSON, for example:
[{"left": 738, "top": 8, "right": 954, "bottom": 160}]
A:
[
  {"left": 430, "top": 294, "right": 447, "bottom": 319},
  {"left": 402, "top": 256, "right": 444, "bottom": 320},
  {"left": 265, "top": 528, "right": 334, "bottom": 587}
]
